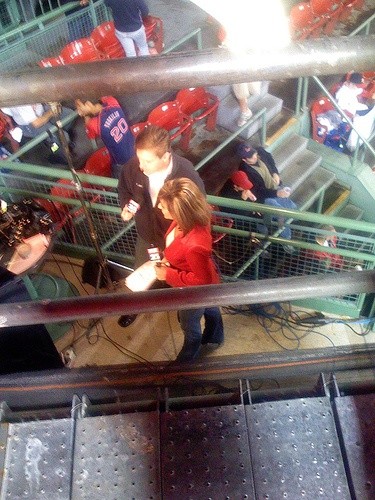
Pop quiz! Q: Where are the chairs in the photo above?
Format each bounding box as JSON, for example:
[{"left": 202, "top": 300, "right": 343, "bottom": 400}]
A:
[
  {"left": 290, "top": 0, "right": 375, "bottom": 142},
  {"left": 0, "top": 16, "right": 233, "bottom": 243}
]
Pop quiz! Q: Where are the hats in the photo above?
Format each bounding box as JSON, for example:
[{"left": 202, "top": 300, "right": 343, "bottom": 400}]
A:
[
  {"left": 231, "top": 171, "right": 254, "bottom": 190},
  {"left": 350, "top": 73, "right": 364, "bottom": 84},
  {"left": 240, "top": 143, "right": 257, "bottom": 158}
]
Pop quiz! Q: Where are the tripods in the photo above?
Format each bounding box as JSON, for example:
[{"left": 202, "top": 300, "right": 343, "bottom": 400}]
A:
[{"left": 51, "top": 102, "right": 135, "bottom": 323}]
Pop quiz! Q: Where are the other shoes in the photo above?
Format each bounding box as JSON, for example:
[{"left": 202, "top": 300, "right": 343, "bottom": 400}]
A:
[
  {"left": 164, "top": 358, "right": 204, "bottom": 370},
  {"left": 198, "top": 339, "right": 224, "bottom": 354},
  {"left": 254, "top": 249, "right": 270, "bottom": 259},
  {"left": 280, "top": 243, "right": 296, "bottom": 254},
  {"left": 237, "top": 109, "right": 253, "bottom": 127},
  {"left": 48, "top": 156, "right": 69, "bottom": 165}
]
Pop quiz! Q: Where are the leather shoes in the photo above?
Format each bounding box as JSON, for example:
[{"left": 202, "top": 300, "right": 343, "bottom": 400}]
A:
[{"left": 116, "top": 313, "right": 137, "bottom": 327}]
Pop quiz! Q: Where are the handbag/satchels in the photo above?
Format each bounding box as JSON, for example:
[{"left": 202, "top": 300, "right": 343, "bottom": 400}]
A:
[{"left": 82, "top": 258, "right": 113, "bottom": 288}]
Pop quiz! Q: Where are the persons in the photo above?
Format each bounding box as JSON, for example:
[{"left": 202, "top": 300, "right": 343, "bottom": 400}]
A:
[
  {"left": 105, "top": 0, "right": 149, "bottom": 57},
  {"left": 237, "top": 143, "right": 300, "bottom": 257},
  {"left": 218, "top": 171, "right": 273, "bottom": 259},
  {"left": 336, "top": 75, "right": 372, "bottom": 154},
  {"left": 77, "top": 97, "right": 134, "bottom": 178},
  {"left": 154, "top": 176, "right": 224, "bottom": 361},
  {"left": 305, "top": 224, "right": 363, "bottom": 301},
  {"left": 221, "top": 36, "right": 261, "bottom": 129},
  {"left": 1, "top": 101, "right": 77, "bottom": 168},
  {"left": 116, "top": 126, "right": 207, "bottom": 327},
  {"left": 63, "top": 0, "right": 99, "bottom": 43},
  {"left": 0, "top": 196, "right": 76, "bottom": 372}
]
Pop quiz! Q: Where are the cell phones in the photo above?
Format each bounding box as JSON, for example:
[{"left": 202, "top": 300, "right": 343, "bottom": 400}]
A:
[
  {"left": 127, "top": 198, "right": 140, "bottom": 215},
  {"left": 146, "top": 246, "right": 161, "bottom": 261}
]
